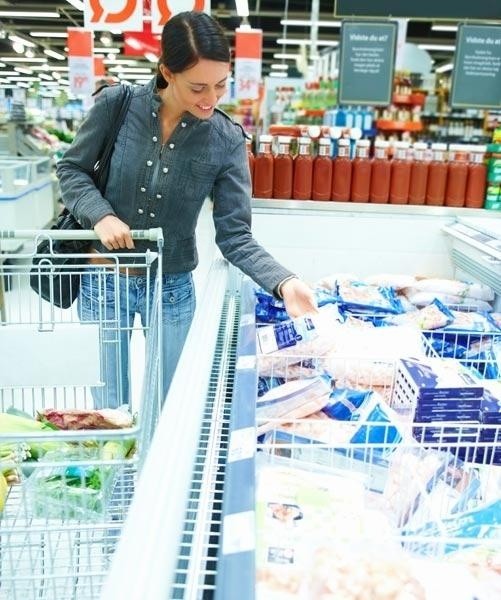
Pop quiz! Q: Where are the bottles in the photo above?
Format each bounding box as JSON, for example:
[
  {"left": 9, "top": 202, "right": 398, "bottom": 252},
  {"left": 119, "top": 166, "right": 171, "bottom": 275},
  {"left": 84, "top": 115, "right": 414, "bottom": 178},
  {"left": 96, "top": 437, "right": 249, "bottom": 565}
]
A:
[
  {"left": 324, "top": 105, "right": 375, "bottom": 133},
  {"left": 246, "top": 124, "right": 487, "bottom": 207}
]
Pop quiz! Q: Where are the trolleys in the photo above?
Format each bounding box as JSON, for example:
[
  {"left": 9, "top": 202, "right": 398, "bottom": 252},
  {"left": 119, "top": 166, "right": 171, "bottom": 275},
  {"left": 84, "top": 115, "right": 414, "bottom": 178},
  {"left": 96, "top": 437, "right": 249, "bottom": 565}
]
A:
[{"left": 1, "top": 227, "right": 164, "bottom": 600}]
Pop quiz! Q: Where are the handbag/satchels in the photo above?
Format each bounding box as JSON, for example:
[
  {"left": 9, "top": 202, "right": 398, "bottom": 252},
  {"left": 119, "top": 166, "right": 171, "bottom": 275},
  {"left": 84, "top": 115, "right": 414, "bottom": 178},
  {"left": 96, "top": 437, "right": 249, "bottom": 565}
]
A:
[{"left": 31, "top": 197, "right": 92, "bottom": 310}]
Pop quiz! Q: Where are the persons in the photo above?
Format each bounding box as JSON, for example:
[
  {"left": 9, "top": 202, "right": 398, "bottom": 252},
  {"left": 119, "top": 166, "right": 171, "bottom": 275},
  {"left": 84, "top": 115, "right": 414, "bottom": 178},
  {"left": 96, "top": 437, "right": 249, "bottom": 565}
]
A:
[{"left": 57, "top": 12, "right": 320, "bottom": 556}]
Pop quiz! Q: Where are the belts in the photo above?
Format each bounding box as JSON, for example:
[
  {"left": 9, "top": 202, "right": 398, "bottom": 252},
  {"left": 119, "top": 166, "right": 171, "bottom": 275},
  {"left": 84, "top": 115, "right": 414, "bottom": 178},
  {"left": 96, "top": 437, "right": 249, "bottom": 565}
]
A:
[{"left": 92, "top": 247, "right": 146, "bottom": 277}]
